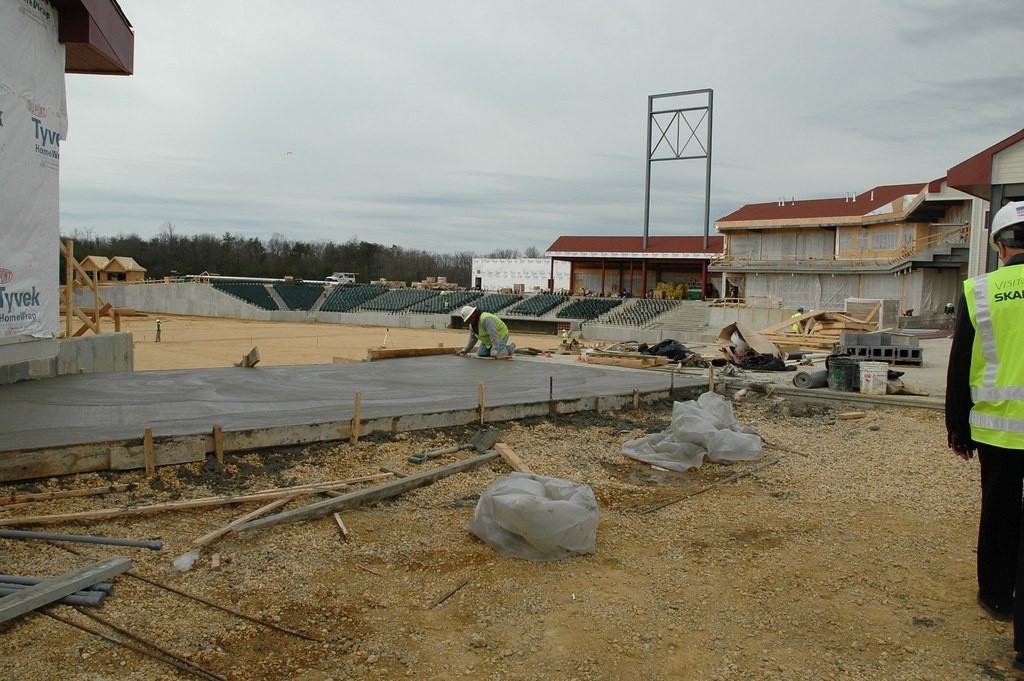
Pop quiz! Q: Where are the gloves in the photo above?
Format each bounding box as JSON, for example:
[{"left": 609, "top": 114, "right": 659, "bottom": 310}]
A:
[
  {"left": 457, "top": 348, "right": 466, "bottom": 353},
  {"left": 490, "top": 350, "right": 498, "bottom": 356}
]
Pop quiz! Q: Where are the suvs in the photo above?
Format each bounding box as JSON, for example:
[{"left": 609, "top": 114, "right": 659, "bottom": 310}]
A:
[{"left": 325, "top": 272, "right": 356, "bottom": 284}]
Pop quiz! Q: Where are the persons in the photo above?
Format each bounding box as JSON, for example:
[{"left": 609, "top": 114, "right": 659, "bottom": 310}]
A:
[
  {"left": 945, "top": 202, "right": 1024, "bottom": 670},
  {"left": 791, "top": 307, "right": 804, "bottom": 330},
  {"left": 155, "top": 319, "right": 162, "bottom": 341},
  {"left": 460, "top": 306, "right": 516, "bottom": 357},
  {"left": 562, "top": 329, "right": 568, "bottom": 344}
]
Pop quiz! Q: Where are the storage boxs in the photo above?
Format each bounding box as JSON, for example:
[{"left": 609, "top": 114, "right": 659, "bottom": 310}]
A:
[
  {"left": 653, "top": 291, "right": 667, "bottom": 299},
  {"left": 426, "top": 277, "right": 447, "bottom": 284},
  {"left": 844, "top": 298, "right": 901, "bottom": 332},
  {"left": 501, "top": 288, "right": 512, "bottom": 295},
  {"left": 514, "top": 284, "right": 525, "bottom": 294},
  {"left": 840, "top": 331, "right": 919, "bottom": 347}
]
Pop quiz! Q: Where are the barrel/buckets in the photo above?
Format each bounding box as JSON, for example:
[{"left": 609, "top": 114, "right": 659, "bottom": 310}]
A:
[
  {"left": 859, "top": 361, "right": 888, "bottom": 395},
  {"left": 828, "top": 357, "right": 856, "bottom": 392}
]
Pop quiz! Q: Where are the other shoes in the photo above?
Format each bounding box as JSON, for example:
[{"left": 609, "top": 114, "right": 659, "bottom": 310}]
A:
[
  {"left": 1012, "top": 650, "right": 1024, "bottom": 671},
  {"left": 507, "top": 342, "right": 516, "bottom": 356},
  {"left": 975, "top": 588, "right": 1013, "bottom": 623}
]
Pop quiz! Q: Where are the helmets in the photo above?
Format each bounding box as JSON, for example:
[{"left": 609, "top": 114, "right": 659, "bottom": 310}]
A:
[
  {"left": 563, "top": 329, "right": 566, "bottom": 332},
  {"left": 798, "top": 308, "right": 804, "bottom": 313},
  {"left": 989, "top": 201, "right": 1024, "bottom": 252},
  {"left": 460, "top": 306, "right": 478, "bottom": 322}
]
